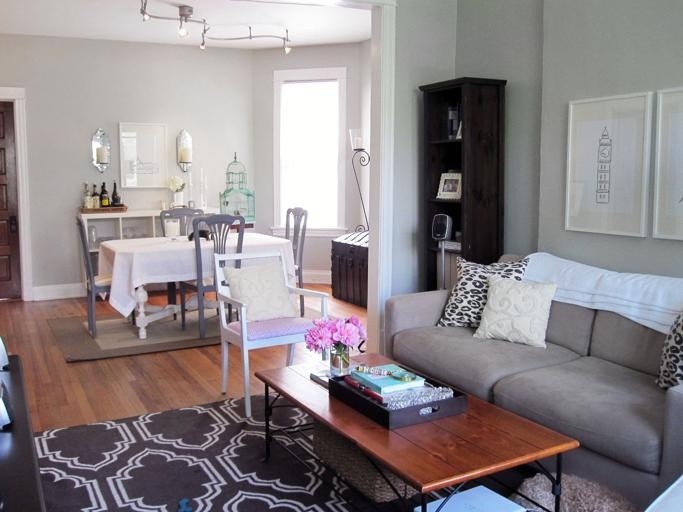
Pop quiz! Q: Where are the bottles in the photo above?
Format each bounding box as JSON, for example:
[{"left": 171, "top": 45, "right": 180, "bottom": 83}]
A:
[
  {"left": 83, "top": 181, "right": 91, "bottom": 209},
  {"left": 112, "top": 182, "right": 117, "bottom": 203},
  {"left": 92, "top": 184, "right": 100, "bottom": 209},
  {"left": 101, "top": 182, "right": 109, "bottom": 206}
]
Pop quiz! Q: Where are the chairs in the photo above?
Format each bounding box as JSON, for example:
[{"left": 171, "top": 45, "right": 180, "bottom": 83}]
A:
[
  {"left": 160, "top": 207, "right": 219, "bottom": 321},
  {"left": 212, "top": 248, "right": 329, "bottom": 419},
  {"left": 177, "top": 213, "right": 245, "bottom": 339},
  {"left": 75, "top": 215, "right": 138, "bottom": 339},
  {"left": 285, "top": 207, "right": 307, "bottom": 317}
]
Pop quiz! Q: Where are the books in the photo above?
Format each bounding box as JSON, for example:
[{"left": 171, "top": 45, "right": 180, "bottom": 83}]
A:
[
  {"left": 350, "top": 364, "right": 424, "bottom": 394},
  {"left": 345, "top": 376, "right": 433, "bottom": 405}
]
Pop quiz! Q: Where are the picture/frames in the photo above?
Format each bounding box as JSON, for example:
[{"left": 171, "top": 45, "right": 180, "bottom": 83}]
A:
[
  {"left": 434, "top": 172, "right": 461, "bottom": 199},
  {"left": 563, "top": 91, "right": 653, "bottom": 239},
  {"left": 118, "top": 122, "right": 168, "bottom": 189},
  {"left": 651, "top": 86, "right": 682, "bottom": 240}
]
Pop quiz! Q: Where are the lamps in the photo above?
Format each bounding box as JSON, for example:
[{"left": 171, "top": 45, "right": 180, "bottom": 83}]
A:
[
  {"left": 142, "top": 12, "right": 150, "bottom": 21},
  {"left": 178, "top": 19, "right": 186, "bottom": 36},
  {"left": 199, "top": 34, "right": 205, "bottom": 50},
  {"left": 348, "top": 126, "right": 370, "bottom": 231},
  {"left": 282, "top": 38, "right": 292, "bottom": 54}
]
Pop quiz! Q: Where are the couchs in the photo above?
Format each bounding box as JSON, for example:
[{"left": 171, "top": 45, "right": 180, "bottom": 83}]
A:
[{"left": 385, "top": 253, "right": 683, "bottom": 508}]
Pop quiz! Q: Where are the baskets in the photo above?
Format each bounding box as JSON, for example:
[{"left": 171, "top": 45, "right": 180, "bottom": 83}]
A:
[{"left": 312, "top": 420, "right": 418, "bottom": 503}]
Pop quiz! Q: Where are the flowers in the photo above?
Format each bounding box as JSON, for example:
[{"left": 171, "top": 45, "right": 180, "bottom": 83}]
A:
[
  {"left": 303, "top": 313, "right": 367, "bottom": 372},
  {"left": 166, "top": 175, "right": 185, "bottom": 192}
]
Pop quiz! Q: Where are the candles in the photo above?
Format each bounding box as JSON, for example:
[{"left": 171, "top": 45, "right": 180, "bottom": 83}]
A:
[
  {"left": 97, "top": 147, "right": 107, "bottom": 163},
  {"left": 180, "top": 148, "right": 192, "bottom": 162}
]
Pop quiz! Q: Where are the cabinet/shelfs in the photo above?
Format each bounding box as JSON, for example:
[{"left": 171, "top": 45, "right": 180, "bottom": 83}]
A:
[
  {"left": 417, "top": 77, "right": 507, "bottom": 293},
  {"left": 77, "top": 210, "right": 165, "bottom": 289}
]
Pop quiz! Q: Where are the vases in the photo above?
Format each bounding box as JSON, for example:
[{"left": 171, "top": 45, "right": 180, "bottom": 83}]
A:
[
  {"left": 329, "top": 341, "right": 349, "bottom": 377},
  {"left": 173, "top": 191, "right": 184, "bottom": 208}
]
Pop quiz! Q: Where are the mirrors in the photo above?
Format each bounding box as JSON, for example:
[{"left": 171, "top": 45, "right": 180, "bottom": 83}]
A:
[
  {"left": 176, "top": 128, "right": 192, "bottom": 172},
  {"left": 91, "top": 127, "right": 111, "bottom": 173}
]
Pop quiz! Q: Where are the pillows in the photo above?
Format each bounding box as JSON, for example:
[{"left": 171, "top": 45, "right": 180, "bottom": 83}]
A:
[
  {"left": 653, "top": 312, "right": 682, "bottom": 389},
  {"left": 471, "top": 275, "right": 556, "bottom": 350},
  {"left": 433, "top": 255, "right": 529, "bottom": 330},
  {"left": 223, "top": 262, "right": 297, "bottom": 324}
]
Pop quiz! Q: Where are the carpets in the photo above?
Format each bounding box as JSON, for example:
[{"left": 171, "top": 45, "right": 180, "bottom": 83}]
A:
[
  {"left": 313, "top": 420, "right": 643, "bottom": 512},
  {"left": 47, "top": 300, "right": 339, "bottom": 363},
  {"left": 33, "top": 390, "right": 358, "bottom": 512}
]
